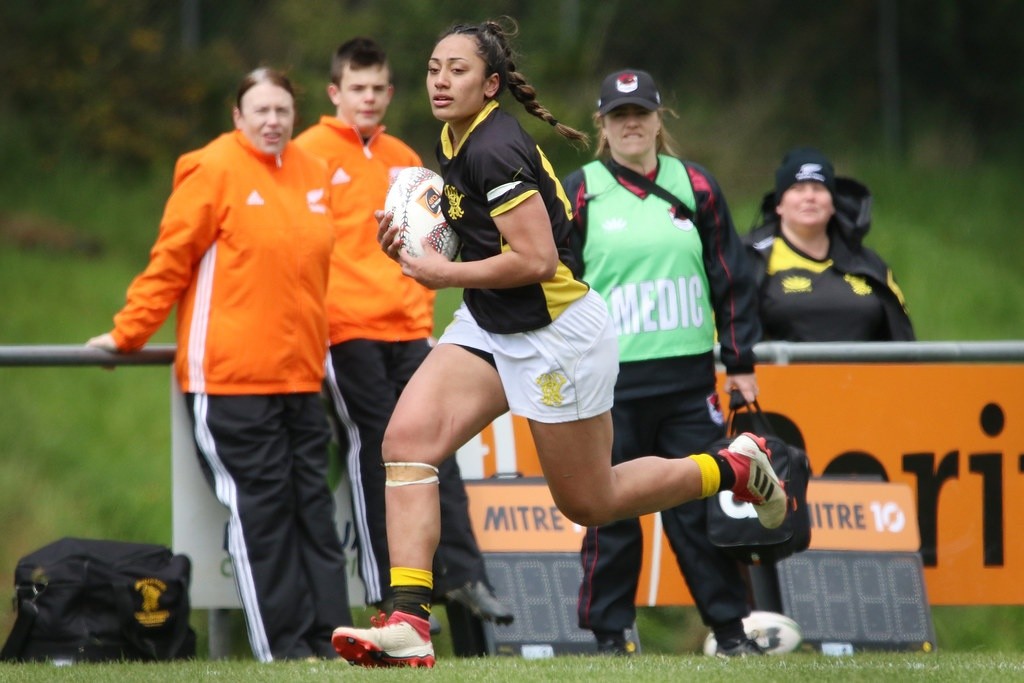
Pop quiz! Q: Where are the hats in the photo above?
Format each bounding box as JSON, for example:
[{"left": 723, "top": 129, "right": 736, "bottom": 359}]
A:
[
  {"left": 600, "top": 68, "right": 659, "bottom": 116},
  {"left": 773, "top": 147, "right": 834, "bottom": 202}
]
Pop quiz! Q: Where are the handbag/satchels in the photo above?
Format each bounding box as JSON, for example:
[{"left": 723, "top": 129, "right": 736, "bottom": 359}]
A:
[
  {"left": 14, "top": 536, "right": 197, "bottom": 662},
  {"left": 705, "top": 398, "right": 813, "bottom": 559}
]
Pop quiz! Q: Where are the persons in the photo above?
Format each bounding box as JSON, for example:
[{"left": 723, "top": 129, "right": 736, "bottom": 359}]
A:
[
  {"left": 86, "top": 65, "right": 354, "bottom": 666},
  {"left": 556, "top": 69, "right": 772, "bottom": 663},
  {"left": 329, "top": 15, "right": 799, "bottom": 670},
  {"left": 742, "top": 151, "right": 918, "bottom": 341},
  {"left": 294, "top": 38, "right": 517, "bottom": 637}
]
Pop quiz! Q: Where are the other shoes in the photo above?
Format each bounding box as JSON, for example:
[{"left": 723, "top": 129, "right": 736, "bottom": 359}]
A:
[{"left": 447, "top": 580, "right": 512, "bottom": 626}]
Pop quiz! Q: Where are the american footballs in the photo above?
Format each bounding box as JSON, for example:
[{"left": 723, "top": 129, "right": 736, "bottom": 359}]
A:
[
  {"left": 382, "top": 166, "right": 461, "bottom": 264},
  {"left": 702, "top": 610, "right": 803, "bottom": 655}
]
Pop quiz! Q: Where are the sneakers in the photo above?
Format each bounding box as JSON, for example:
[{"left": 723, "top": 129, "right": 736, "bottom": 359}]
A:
[
  {"left": 721, "top": 431, "right": 789, "bottom": 529},
  {"left": 717, "top": 632, "right": 766, "bottom": 660},
  {"left": 330, "top": 610, "right": 435, "bottom": 669}
]
